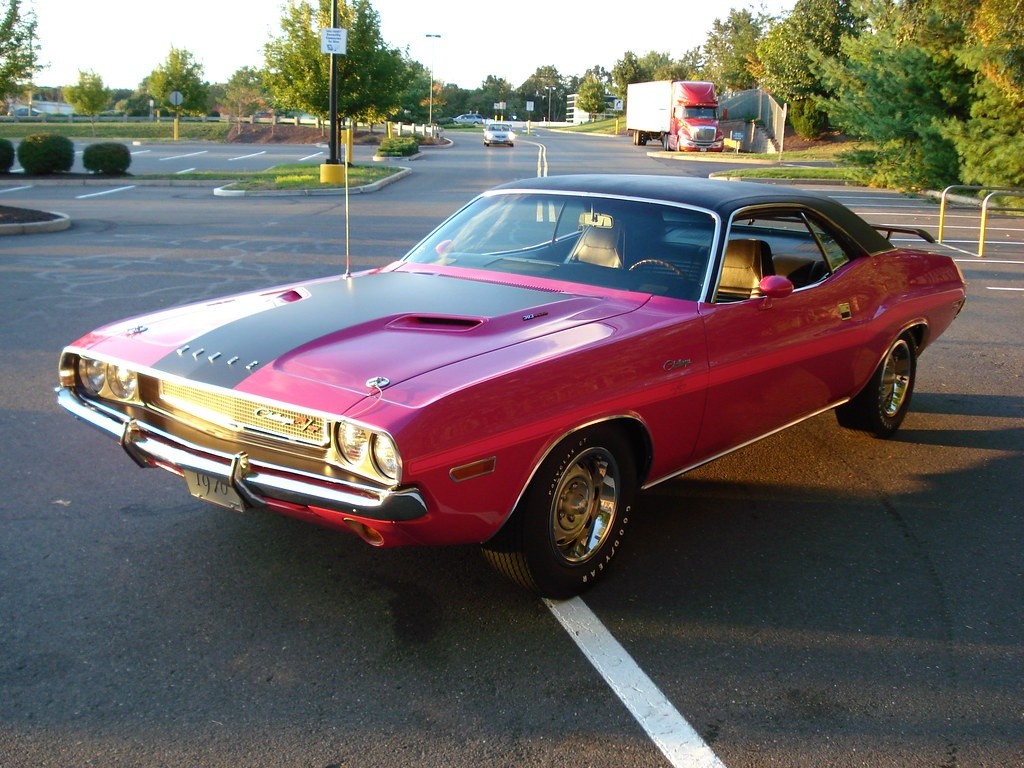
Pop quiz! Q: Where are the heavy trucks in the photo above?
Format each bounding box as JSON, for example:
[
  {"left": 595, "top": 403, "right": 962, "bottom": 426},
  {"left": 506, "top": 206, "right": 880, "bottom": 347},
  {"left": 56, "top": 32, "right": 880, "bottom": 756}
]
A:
[{"left": 627, "top": 80, "right": 728, "bottom": 152}]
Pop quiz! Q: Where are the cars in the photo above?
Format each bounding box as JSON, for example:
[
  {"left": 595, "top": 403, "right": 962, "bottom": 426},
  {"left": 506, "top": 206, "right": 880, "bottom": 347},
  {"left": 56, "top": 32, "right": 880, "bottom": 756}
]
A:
[
  {"left": 48, "top": 175, "right": 970, "bottom": 600},
  {"left": 482, "top": 124, "right": 515, "bottom": 147},
  {"left": 8, "top": 108, "right": 42, "bottom": 117},
  {"left": 453, "top": 114, "right": 483, "bottom": 126}
]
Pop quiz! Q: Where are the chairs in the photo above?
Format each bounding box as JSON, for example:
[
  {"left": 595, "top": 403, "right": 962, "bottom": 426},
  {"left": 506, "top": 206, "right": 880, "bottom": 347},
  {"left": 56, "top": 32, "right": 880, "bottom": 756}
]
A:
[
  {"left": 718, "top": 237, "right": 775, "bottom": 300},
  {"left": 565, "top": 216, "right": 627, "bottom": 271}
]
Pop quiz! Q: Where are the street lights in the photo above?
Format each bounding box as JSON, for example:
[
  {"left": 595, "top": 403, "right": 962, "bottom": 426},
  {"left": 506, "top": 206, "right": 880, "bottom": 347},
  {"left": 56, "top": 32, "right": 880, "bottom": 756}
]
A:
[
  {"left": 426, "top": 35, "right": 442, "bottom": 125},
  {"left": 545, "top": 85, "right": 556, "bottom": 122}
]
